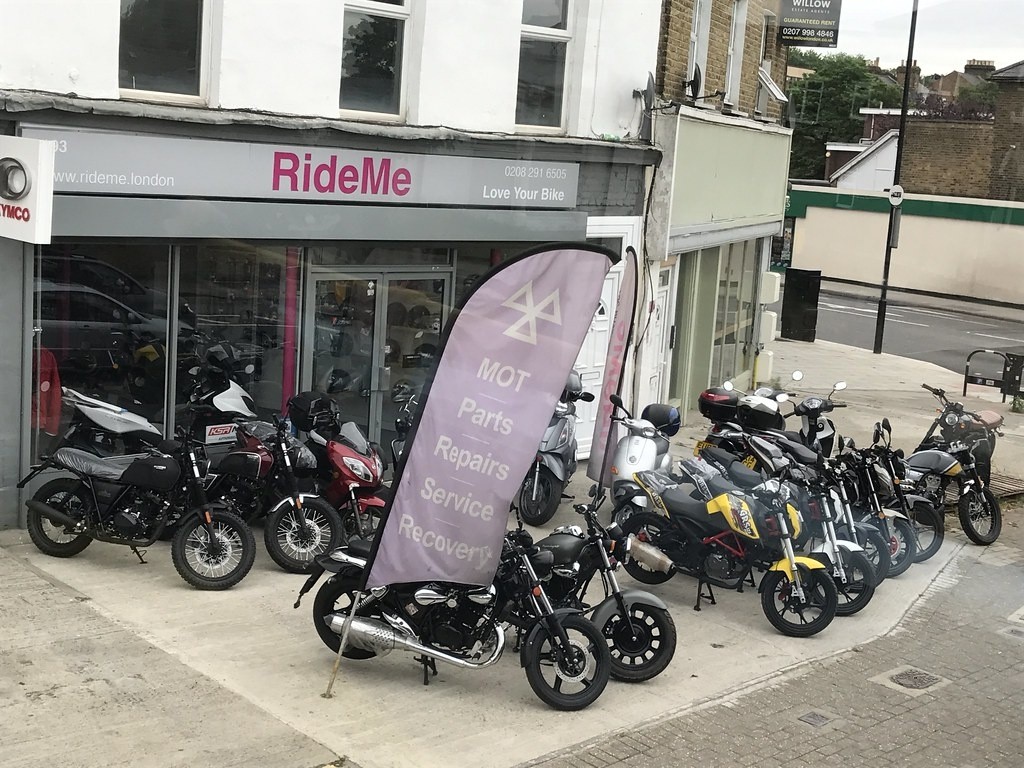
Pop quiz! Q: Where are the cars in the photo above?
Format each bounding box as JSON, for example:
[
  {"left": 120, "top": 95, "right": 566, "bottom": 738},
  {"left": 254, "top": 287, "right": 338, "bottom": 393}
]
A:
[
  {"left": 335, "top": 258, "right": 481, "bottom": 329},
  {"left": 32, "top": 281, "right": 195, "bottom": 380},
  {"left": 34, "top": 254, "right": 197, "bottom": 327}
]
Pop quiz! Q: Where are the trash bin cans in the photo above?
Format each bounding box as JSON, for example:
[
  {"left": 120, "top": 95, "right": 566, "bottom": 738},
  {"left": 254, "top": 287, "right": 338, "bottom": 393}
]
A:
[{"left": 781, "top": 268, "right": 821, "bottom": 343}]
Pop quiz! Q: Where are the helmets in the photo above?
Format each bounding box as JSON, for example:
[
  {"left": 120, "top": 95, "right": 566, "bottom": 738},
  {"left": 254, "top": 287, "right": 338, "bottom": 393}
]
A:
[{"left": 319, "top": 273, "right": 479, "bottom": 403}]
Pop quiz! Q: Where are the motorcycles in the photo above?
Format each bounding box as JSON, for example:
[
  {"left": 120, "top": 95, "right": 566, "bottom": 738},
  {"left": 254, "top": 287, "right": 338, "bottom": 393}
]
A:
[
  {"left": 390, "top": 394, "right": 419, "bottom": 473},
  {"left": 912, "top": 382, "right": 1006, "bottom": 491},
  {"left": 519, "top": 369, "right": 595, "bottom": 527},
  {"left": 687, "top": 370, "right": 804, "bottom": 458},
  {"left": 39, "top": 339, "right": 258, "bottom": 472},
  {"left": 229, "top": 391, "right": 392, "bottom": 546},
  {"left": 161, "top": 395, "right": 344, "bottom": 575},
  {"left": 606, "top": 394, "right": 681, "bottom": 525},
  {"left": 294, "top": 504, "right": 677, "bottom": 711},
  {"left": 612, "top": 417, "right": 1002, "bottom": 637},
  {"left": 15, "top": 426, "right": 256, "bottom": 591}
]
